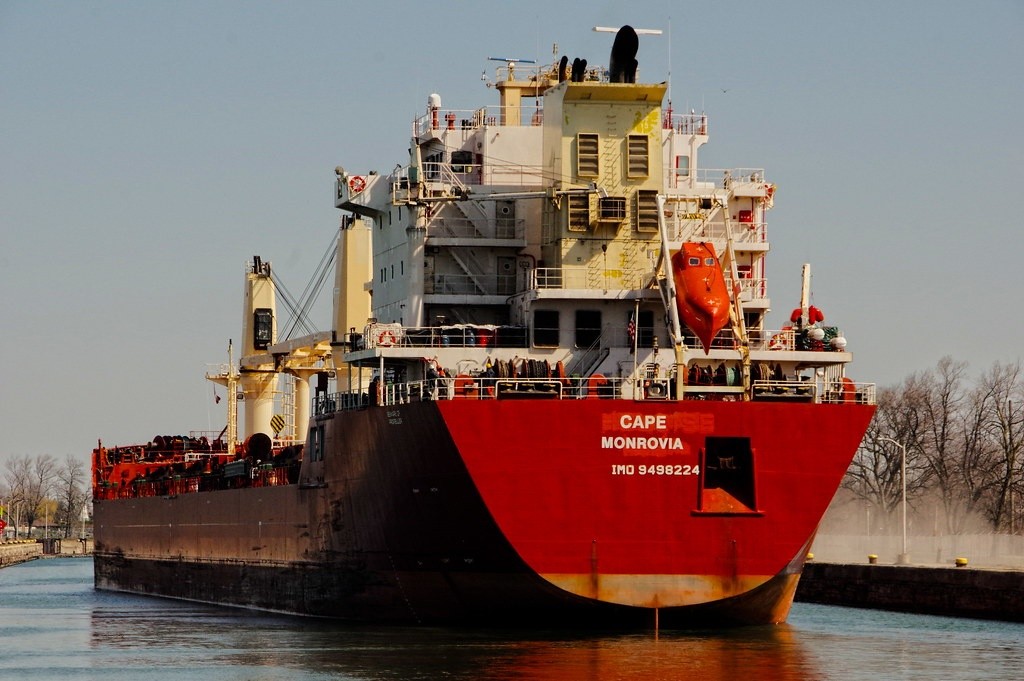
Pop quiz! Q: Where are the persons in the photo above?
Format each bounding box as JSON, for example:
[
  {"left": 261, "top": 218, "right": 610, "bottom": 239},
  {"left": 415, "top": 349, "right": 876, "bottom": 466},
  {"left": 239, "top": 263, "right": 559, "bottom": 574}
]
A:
[{"left": 427, "top": 364, "right": 451, "bottom": 391}]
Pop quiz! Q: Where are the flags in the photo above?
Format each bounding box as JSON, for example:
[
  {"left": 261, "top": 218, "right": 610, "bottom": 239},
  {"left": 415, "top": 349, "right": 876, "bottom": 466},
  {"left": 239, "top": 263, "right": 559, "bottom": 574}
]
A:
[{"left": 628, "top": 314, "right": 635, "bottom": 353}]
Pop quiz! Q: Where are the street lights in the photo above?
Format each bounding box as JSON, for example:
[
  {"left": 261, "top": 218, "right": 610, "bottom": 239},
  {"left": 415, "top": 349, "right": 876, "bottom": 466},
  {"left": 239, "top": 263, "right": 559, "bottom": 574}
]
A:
[
  {"left": 876, "top": 437, "right": 909, "bottom": 568},
  {"left": 6, "top": 498, "right": 20, "bottom": 539},
  {"left": 16, "top": 500, "right": 29, "bottom": 539}
]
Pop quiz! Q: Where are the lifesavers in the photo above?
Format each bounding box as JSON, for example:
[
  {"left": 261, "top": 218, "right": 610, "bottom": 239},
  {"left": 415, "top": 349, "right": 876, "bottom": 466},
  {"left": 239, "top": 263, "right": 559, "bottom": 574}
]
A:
[
  {"left": 379, "top": 329, "right": 396, "bottom": 347},
  {"left": 351, "top": 176, "right": 366, "bottom": 192}
]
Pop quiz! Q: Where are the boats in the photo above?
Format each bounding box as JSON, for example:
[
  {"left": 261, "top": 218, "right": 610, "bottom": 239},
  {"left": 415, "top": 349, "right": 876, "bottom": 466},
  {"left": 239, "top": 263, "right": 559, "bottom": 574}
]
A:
[
  {"left": 91, "top": 26, "right": 879, "bottom": 640},
  {"left": 671, "top": 240, "right": 730, "bottom": 355}
]
